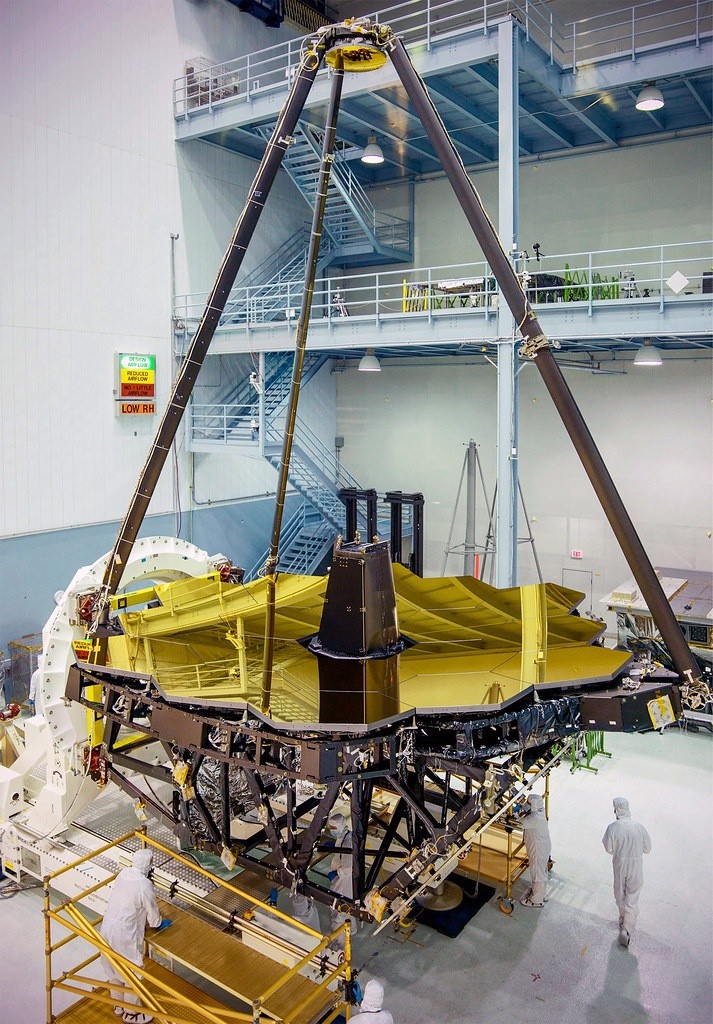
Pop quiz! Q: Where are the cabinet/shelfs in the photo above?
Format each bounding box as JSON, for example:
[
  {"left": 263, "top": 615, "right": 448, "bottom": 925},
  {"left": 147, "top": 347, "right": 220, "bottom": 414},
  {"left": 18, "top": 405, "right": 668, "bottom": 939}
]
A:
[{"left": 41, "top": 825, "right": 353, "bottom": 1024}]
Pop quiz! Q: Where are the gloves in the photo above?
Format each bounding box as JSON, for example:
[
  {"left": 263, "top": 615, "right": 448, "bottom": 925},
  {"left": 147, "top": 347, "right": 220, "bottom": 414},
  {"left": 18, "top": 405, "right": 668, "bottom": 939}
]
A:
[
  {"left": 328, "top": 871, "right": 337, "bottom": 881},
  {"left": 353, "top": 981, "right": 363, "bottom": 1004},
  {"left": 324, "top": 841, "right": 335, "bottom": 847},
  {"left": 157, "top": 919, "right": 173, "bottom": 929},
  {"left": 513, "top": 805, "right": 520, "bottom": 812},
  {"left": 270, "top": 888, "right": 278, "bottom": 902}
]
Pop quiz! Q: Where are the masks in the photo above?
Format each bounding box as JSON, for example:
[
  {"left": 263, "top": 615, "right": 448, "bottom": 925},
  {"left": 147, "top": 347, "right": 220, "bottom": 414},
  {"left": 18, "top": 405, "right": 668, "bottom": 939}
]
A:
[
  {"left": 523, "top": 802, "right": 531, "bottom": 812},
  {"left": 329, "top": 829, "right": 338, "bottom": 837}
]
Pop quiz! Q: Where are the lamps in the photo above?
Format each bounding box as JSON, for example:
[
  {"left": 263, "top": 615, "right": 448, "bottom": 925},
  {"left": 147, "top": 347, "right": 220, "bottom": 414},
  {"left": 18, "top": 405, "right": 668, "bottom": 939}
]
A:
[
  {"left": 635, "top": 80, "right": 664, "bottom": 111},
  {"left": 633, "top": 337, "right": 663, "bottom": 366},
  {"left": 361, "top": 136, "right": 384, "bottom": 164},
  {"left": 357, "top": 348, "right": 381, "bottom": 371}
]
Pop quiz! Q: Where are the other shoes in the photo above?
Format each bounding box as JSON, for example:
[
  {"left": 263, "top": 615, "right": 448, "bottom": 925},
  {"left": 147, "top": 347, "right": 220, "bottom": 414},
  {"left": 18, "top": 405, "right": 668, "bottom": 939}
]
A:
[
  {"left": 521, "top": 898, "right": 545, "bottom": 907},
  {"left": 113, "top": 1005, "right": 124, "bottom": 1015},
  {"left": 122, "top": 1013, "right": 154, "bottom": 1024},
  {"left": 528, "top": 888, "right": 549, "bottom": 902},
  {"left": 619, "top": 930, "right": 630, "bottom": 948}
]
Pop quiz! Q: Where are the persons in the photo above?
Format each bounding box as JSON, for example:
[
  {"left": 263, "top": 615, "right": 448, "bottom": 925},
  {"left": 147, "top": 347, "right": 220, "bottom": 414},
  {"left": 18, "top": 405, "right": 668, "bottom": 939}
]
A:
[
  {"left": 28, "top": 655, "right": 42, "bottom": 716},
  {"left": 100, "top": 849, "right": 173, "bottom": 1024},
  {"left": 512, "top": 794, "right": 551, "bottom": 906},
  {"left": 328, "top": 813, "right": 357, "bottom": 935},
  {"left": 602, "top": 797, "right": 652, "bottom": 948},
  {"left": 347, "top": 980, "right": 393, "bottom": 1024},
  {"left": 291, "top": 894, "right": 320, "bottom": 931}
]
